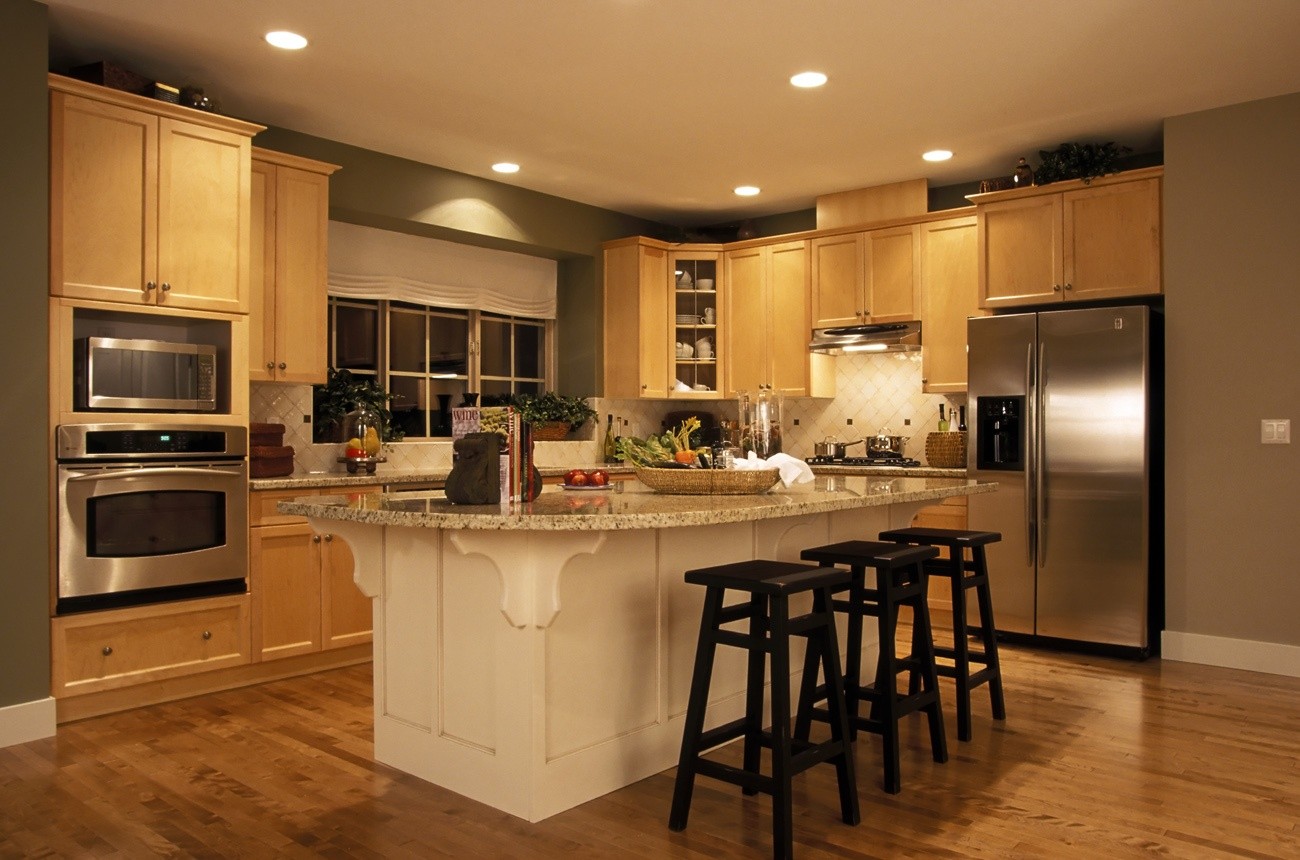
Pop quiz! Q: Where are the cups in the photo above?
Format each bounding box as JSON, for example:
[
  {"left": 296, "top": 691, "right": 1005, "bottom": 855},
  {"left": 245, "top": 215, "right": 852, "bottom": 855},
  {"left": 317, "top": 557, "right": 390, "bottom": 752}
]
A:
[
  {"left": 676, "top": 341, "right": 694, "bottom": 358},
  {"left": 695, "top": 445, "right": 741, "bottom": 470},
  {"left": 700, "top": 306, "right": 716, "bottom": 324},
  {"left": 697, "top": 279, "right": 713, "bottom": 289},
  {"left": 693, "top": 384, "right": 710, "bottom": 391},
  {"left": 676, "top": 270, "right": 691, "bottom": 283},
  {"left": 697, "top": 336, "right": 714, "bottom": 361}
]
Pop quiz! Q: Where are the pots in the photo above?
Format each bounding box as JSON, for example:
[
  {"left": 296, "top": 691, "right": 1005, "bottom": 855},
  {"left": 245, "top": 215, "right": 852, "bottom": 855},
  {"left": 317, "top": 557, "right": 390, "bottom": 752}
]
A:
[
  {"left": 814, "top": 436, "right": 864, "bottom": 458},
  {"left": 865, "top": 427, "right": 910, "bottom": 458}
]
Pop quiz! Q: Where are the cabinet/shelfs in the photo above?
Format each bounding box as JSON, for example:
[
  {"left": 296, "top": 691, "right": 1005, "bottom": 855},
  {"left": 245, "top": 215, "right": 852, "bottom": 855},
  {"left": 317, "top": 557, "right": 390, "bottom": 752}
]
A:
[
  {"left": 898, "top": 494, "right": 969, "bottom": 632},
  {"left": 47, "top": 71, "right": 269, "bottom": 315},
  {"left": 668, "top": 242, "right": 724, "bottom": 400},
  {"left": 248, "top": 145, "right": 344, "bottom": 386},
  {"left": 601, "top": 235, "right": 670, "bottom": 400},
  {"left": 723, "top": 231, "right": 836, "bottom": 398},
  {"left": 963, "top": 163, "right": 1167, "bottom": 312},
  {"left": 920, "top": 205, "right": 1012, "bottom": 394},
  {"left": 249, "top": 484, "right": 383, "bottom": 684},
  {"left": 811, "top": 214, "right": 922, "bottom": 330},
  {"left": 51, "top": 591, "right": 254, "bottom": 726}
]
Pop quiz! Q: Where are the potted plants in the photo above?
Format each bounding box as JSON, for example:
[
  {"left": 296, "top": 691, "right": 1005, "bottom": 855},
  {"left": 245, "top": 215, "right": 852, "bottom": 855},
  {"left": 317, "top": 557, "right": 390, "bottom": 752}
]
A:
[{"left": 508, "top": 389, "right": 601, "bottom": 441}]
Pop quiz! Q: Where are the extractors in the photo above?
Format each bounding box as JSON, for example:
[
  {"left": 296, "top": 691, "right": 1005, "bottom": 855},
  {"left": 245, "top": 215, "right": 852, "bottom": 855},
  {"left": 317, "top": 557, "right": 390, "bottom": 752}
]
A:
[{"left": 808, "top": 320, "right": 922, "bottom": 357}]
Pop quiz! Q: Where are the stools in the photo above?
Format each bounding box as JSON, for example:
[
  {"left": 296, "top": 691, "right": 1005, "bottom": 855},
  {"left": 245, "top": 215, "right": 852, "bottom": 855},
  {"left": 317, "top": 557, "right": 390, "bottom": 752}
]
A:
[
  {"left": 667, "top": 558, "right": 864, "bottom": 860},
  {"left": 868, "top": 526, "right": 1007, "bottom": 742},
  {"left": 792, "top": 539, "right": 951, "bottom": 795}
]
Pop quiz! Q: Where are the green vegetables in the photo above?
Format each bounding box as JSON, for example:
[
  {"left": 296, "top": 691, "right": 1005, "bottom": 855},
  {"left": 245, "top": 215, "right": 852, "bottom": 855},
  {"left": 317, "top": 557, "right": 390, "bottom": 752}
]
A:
[{"left": 613, "top": 415, "right": 702, "bottom": 468}]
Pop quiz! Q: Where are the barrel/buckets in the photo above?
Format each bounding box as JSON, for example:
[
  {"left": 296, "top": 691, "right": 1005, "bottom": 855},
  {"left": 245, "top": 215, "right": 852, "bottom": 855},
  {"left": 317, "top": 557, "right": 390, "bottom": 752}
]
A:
[{"left": 736, "top": 388, "right": 781, "bottom": 461}]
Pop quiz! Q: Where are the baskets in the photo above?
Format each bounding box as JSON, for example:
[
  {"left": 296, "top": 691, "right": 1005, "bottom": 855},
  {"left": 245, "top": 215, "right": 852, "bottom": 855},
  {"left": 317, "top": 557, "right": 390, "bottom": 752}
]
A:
[
  {"left": 634, "top": 464, "right": 781, "bottom": 495},
  {"left": 925, "top": 431, "right": 968, "bottom": 469}
]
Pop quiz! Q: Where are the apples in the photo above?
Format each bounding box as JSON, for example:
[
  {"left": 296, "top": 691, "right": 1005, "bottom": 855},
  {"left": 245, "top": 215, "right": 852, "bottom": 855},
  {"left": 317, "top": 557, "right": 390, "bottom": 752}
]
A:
[{"left": 563, "top": 469, "right": 610, "bottom": 486}]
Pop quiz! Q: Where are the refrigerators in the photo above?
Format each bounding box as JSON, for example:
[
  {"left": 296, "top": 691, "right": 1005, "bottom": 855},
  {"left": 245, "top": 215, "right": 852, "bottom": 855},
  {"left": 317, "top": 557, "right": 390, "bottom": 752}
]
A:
[{"left": 964, "top": 301, "right": 1164, "bottom": 662}]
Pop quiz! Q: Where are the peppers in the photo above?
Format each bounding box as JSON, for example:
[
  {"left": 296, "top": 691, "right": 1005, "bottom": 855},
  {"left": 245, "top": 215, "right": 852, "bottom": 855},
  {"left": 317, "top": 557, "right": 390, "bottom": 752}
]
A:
[{"left": 675, "top": 448, "right": 696, "bottom": 464}]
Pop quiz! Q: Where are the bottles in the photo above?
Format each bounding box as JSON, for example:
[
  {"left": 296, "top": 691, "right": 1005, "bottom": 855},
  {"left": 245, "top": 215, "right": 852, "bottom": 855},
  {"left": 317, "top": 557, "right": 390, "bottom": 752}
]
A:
[
  {"left": 604, "top": 414, "right": 616, "bottom": 463},
  {"left": 938, "top": 404, "right": 967, "bottom": 432},
  {"left": 736, "top": 219, "right": 758, "bottom": 240},
  {"left": 615, "top": 417, "right": 625, "bottom": 463},
  {"left": 1014, "top": 158, "right": 1033, "bottom": 188}
]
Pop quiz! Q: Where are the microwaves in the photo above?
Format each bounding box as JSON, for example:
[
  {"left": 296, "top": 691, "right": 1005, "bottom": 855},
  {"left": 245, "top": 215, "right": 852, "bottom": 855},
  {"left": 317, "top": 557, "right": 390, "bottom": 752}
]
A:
[{"left": 73, "top": 335, "right": 218, "bottom": 414}]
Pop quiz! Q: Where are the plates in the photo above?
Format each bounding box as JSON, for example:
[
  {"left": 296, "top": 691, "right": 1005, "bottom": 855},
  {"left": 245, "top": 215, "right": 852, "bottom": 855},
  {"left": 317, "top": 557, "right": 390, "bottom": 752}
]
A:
[
  {"left": 676, "top": 282, "right": 693, "bottom": 289},
  {"left": 676, "top": 315, "right": 701, "bottom": 324},
  {"left": 557, "top": 483, "right": 615, "bottom": 490}
]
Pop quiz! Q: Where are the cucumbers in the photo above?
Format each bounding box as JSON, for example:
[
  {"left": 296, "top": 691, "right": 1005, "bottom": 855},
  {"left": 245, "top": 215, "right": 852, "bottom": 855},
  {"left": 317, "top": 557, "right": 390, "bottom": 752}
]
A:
[{"left": 698, "top": 452, "right": 710, "bottom": 468}]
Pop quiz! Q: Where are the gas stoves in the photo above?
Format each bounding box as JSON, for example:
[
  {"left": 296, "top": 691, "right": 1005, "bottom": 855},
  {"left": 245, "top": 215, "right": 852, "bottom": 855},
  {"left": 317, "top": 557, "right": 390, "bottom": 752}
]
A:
[{"left": 804, "top": 456, "right": 921, "bottom": 467}]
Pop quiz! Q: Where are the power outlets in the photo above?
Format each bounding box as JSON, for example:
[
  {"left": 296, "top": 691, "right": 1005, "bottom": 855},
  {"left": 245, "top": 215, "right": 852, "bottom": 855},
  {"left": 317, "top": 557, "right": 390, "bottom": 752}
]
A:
[{"left": 266, "top": 416, "right": 280, "bottom": 424}]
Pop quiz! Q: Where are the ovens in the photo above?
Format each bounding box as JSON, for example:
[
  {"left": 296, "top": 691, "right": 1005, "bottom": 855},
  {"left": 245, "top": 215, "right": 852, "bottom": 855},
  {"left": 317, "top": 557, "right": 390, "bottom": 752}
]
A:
[{"left": 56, "top": 422, "right": 247, "bottom": 614}]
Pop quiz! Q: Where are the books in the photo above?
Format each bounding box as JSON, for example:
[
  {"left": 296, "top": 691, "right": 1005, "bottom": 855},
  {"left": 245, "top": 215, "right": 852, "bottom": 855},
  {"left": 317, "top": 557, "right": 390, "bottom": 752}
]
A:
[{"left": 451, "top": 407, "right": 534, "bottom": 503}]
